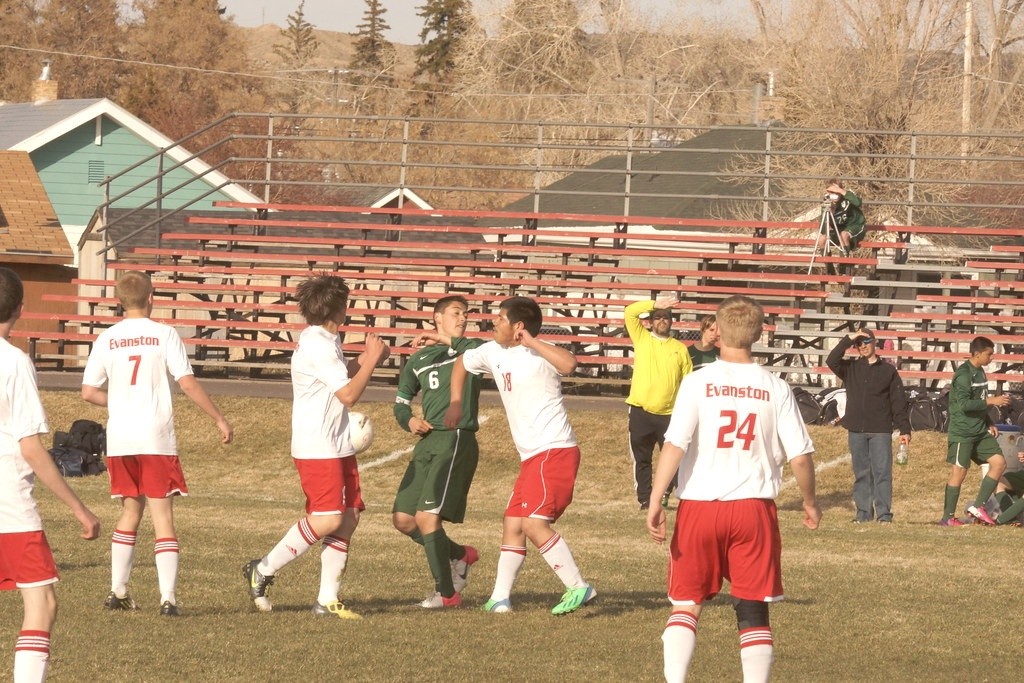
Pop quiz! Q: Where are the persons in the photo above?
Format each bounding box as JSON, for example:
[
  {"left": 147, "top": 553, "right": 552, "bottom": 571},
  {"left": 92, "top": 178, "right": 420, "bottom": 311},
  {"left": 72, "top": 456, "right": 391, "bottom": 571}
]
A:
[
  {"left": 818, "top": 178, "right": 867, "bottom": 276},
  {"left": 938, "top": 336, "right": 1011, "bottom": 525},
  {"left": 624, "top": 297, "right": 679, "bottom": 509},
  {"left": 686, "top": 314, "right": 720, "bottom": 372},
  {"left": 648, "top": 296, "right": 823, "bottom": 683},
  {"left": 826, "top": 328, "right": 912, "bottom": 523},
  {"left": 392, "top": 296, "right": 486, "bottom": 609},
  {"left": 0, "top": 266, "right": 102, "bottom": 682},
  {"left": 81, "top": 271, "right": 233, "bottom": 617},
  {"left": 988, "top": 451, "right": 1024, "bottom": 526},
  {"left": 241, "top": 273, "right": 390, "bottom": 621},
  {"left": 445, "top": 296, "right": 598, "bottom": 618}
]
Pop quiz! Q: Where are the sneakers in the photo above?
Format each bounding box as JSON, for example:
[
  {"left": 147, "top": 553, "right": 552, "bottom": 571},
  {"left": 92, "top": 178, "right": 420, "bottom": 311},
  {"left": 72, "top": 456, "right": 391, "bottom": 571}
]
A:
[
  {"left": 418, "top": 592, "right": 461, "bottom": 609},
  {"left": 967, "top": 505, "right": 995, "bottom": 525},
  {"left": 242, "top": 559, "right": 275, "bottom": 612},
  {"left": 310, "top": 600, "right": 363, "bottom": 620},
  {"left": 160, "top": 600, "right": 178, "bottom": 617},
  {"left": 940, "top": 518, "right": 969, "bottom": 526},
  {"left": 103, "top": 591, "right": 142, "bottom": 612},
  {"left": 551, "top": 583, "right": 597, "bottom": 617},
  {"left": 479, "top": 599, "right": 512, "bottom": 613},
  {"left": 451, "top": 546, "right": 479, "bottom": 592}
]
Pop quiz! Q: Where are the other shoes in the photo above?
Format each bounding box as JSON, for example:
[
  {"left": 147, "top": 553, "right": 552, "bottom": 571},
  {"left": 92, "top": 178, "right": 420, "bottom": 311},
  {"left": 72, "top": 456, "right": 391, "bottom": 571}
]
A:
[
  {"left": 641, "top": 500, "right": 649, "bottom": 510},
  {"left": 661, "top": 493, "right": 669, "bottom": 507}
]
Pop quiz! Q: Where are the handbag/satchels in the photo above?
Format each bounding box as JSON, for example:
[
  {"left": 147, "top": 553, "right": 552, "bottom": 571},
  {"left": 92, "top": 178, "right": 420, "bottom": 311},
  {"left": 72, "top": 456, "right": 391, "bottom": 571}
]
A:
[{"left": 47, "top": 419, "right": 106, "bottom": 478}]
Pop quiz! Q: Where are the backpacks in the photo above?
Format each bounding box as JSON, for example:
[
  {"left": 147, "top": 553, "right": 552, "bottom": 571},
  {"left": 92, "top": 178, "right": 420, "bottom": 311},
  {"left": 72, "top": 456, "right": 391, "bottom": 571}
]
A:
[
  {"left": 791, "top": 386, "right": 846, "bottom": 426},
  {"left": 903, "top": 383, "right": 1024, "bottom": 432}
]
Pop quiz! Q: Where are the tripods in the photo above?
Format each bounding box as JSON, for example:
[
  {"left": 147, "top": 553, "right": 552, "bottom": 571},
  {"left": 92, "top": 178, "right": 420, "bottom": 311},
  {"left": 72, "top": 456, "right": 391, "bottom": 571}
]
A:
[{"left": 804, "top": 202, "right": 848, "bottom": 290}]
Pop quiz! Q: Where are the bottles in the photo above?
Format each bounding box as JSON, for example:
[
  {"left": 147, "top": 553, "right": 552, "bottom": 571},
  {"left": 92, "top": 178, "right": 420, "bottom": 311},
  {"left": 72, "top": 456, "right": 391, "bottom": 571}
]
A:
[{"left": 897, "top": 441, "right": 908, "bottom": 466}]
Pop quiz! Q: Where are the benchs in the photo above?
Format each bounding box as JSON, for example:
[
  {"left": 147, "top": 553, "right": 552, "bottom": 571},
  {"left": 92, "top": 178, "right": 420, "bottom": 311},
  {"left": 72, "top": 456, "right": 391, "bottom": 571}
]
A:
[{"left": 7, "top": 197, "right": 1024, "bottom": 398}]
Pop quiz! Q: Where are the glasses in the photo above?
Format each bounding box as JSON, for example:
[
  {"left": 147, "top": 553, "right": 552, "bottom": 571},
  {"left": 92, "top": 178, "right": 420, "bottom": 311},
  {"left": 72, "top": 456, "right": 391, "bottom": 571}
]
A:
[
  {"left": 652, "top": 315, "right": 670, "bottom": 319},
  {"left": 855, "top": 338, "right": 872, "bottom": 346}
]
